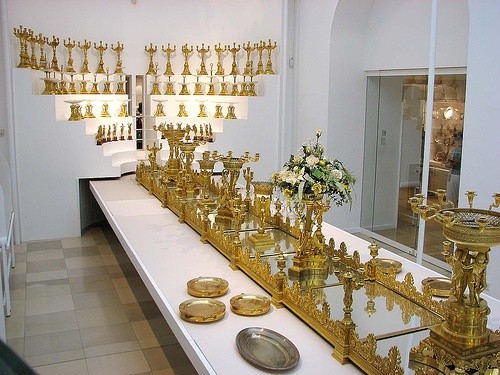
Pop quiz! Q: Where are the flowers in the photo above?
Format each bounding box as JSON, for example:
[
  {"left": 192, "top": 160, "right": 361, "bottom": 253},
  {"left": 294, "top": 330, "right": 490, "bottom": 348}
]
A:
[{"left": 272, "top": 129, "right": 356, "bottom": 200}]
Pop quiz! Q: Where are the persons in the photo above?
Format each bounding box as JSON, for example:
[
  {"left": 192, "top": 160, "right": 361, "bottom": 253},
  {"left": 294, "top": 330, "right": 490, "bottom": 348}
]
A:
[{"left": 441, "top": 131, "right": 463, "bottom": 209}]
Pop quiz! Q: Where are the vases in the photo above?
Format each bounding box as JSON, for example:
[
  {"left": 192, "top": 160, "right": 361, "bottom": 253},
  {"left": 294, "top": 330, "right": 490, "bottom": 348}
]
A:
[{"left": 300, "top": 192, "right": 324, "bottom": 235}]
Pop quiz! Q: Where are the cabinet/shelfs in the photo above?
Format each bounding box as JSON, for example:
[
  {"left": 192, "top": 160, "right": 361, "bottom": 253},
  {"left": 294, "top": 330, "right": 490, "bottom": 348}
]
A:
[
  {"left": 430, "top": 167, "right": 448, "bottom": 194},
  {"left": 13, "top": 67, "right": 139, "bottom": 178},
  {"left": 142, "top": 74, "right": 281, "bottom": 174}
]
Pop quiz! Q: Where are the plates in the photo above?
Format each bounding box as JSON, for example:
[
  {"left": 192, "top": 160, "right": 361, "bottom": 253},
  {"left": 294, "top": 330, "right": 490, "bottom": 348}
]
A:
[
  {"left": 367, "top": 258, "right": 402, "bottom": 274},
  {"left": 186, "top": 276, "right": 229, "bottom": 298},
  {"left": 179, "top": 297, "right": 227, "bottom": 324},
  {"left": 421, "top": 277, "right": 451, "bottom": 297},
  {"left": 236, "top": 327, "right": 300, "bottom": 371},
  {"left": 229, "top": 293, "right": 271, "bottom": 316}
]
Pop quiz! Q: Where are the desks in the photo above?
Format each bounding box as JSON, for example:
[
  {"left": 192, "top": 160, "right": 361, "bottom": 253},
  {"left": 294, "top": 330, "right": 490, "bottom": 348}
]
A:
[{"left": 90, "top": 178, "right": 500, "bottom": 375}]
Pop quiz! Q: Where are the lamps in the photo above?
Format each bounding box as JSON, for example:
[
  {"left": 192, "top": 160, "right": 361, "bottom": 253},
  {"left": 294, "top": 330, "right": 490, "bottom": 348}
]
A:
[{"left": 434, "top": 103, "right": 464, "bottom": 121}]
